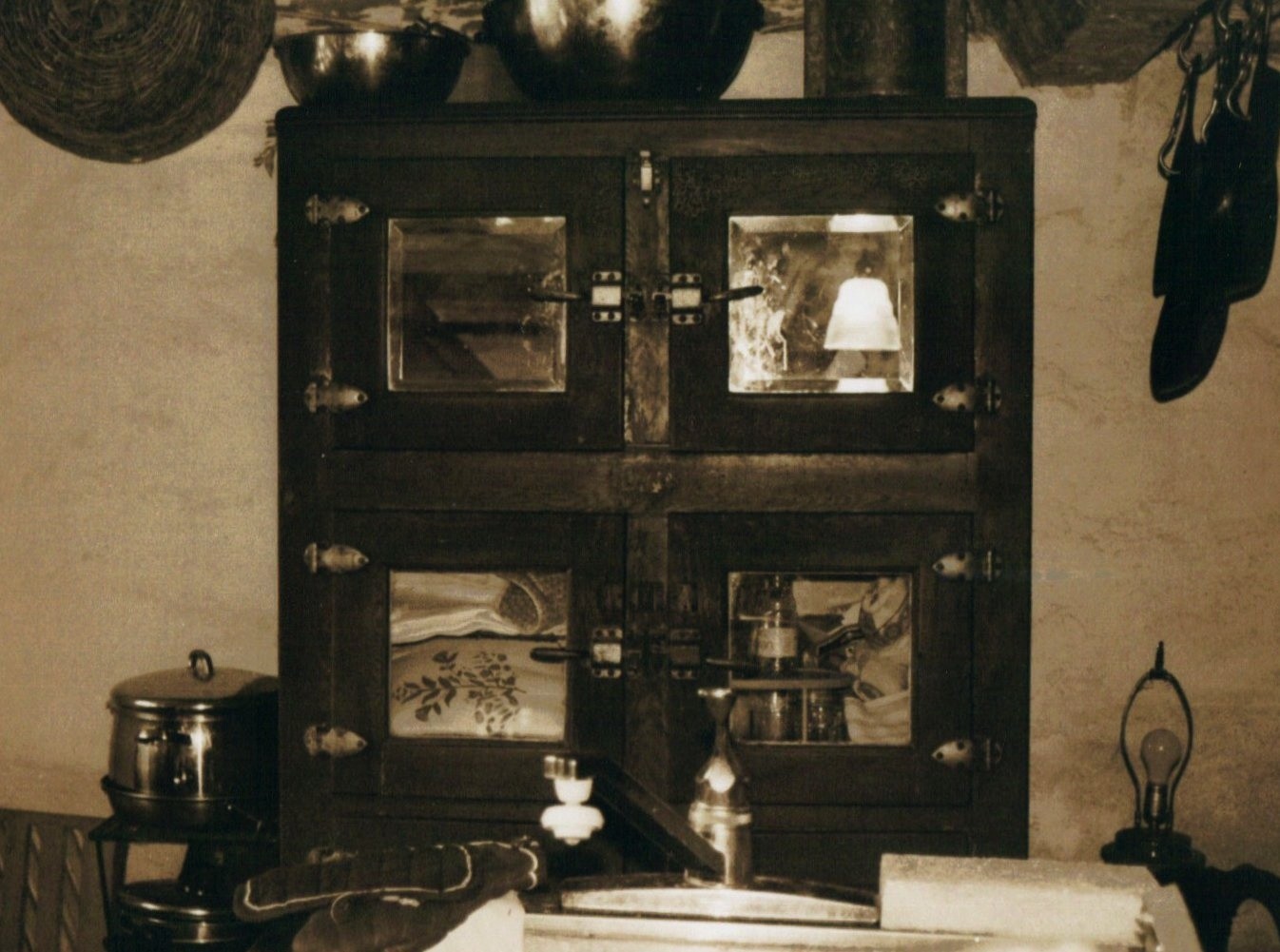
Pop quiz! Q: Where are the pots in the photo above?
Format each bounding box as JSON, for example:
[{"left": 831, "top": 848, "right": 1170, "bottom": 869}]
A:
[
  {"left": 1148, "top": 0, "right": 1280, "bottom": 404},
  {"left": 99, "top": 649, "right": 278, "bottom": 826},
  {"left": 114, "top": 878, "right": 283, "bottom": 952}
]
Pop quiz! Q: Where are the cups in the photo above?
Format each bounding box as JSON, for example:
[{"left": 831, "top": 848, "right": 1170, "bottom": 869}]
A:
[{"left": 790, "top": 675, "right": 836, "bottom": 740}]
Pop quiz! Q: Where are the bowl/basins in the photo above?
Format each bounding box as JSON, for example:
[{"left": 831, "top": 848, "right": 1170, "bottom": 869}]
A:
[
  {"left": 480, "top": 0, "right": 765, "bottom": 106},
  {"left": 275, "top": 23, "right": 475, "bottom": 109}
]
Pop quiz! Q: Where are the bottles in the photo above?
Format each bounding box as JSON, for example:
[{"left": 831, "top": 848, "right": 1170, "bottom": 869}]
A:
[{"left": 750, "top": 575, "right": 800, "bottom": 739}]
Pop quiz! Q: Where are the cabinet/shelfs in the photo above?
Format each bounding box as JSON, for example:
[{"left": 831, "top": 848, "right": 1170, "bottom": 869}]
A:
[{"left": 270, "top": 92, "right": 1031, "bottom": 886}]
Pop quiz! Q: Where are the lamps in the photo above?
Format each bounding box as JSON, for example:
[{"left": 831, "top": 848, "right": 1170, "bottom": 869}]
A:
[{"left": 825, "top": 275, "right": 903, "bottom": 353}]
